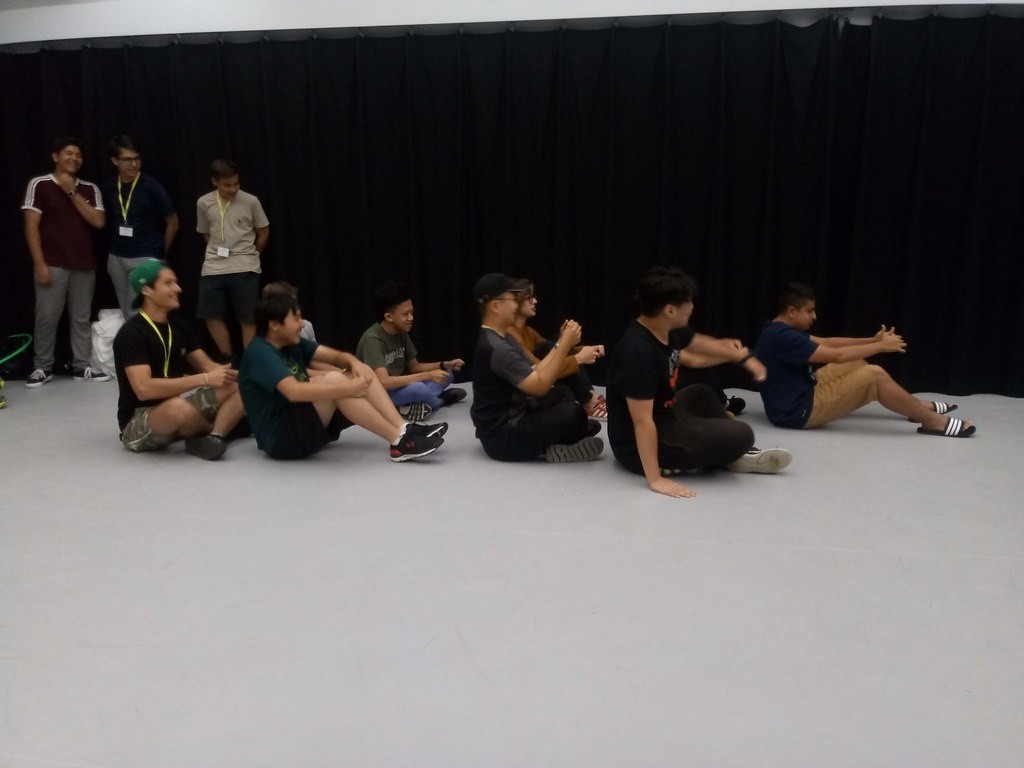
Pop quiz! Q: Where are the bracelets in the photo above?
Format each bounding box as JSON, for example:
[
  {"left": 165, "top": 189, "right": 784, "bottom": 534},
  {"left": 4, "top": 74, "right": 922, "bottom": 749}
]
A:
[
  {"left": 737, "top": 351, "right": 754, "bottom": 366},
  {"left": 440, "top": 361, "right": 445, "bottom": 369},
  {"left": 553, "top": 344, "right": 559, "bottom": 349},
  {"left": 205, "top": 374, "right": 208, "bottom": 382}
]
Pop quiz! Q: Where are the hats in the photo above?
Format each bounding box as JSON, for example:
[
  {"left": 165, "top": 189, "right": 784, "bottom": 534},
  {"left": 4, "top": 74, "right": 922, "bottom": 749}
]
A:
[
  {"left": 474, "top": 274, "right": 526, "bottom": 305},
  {"left": 127, "top": 257, "right": 167, "bottom": 310}
]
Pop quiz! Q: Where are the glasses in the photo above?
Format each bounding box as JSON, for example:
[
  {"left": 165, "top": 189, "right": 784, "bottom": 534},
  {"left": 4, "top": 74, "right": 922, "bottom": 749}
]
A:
[
  {"left": 118, "top": 153, "right": 141, "bottom": 166},
  {"left": 523, "top": 293, "right": 537, "bottom": 303},
  {"left": 492, "top": 294, "right": 522, "bottom": 306}
]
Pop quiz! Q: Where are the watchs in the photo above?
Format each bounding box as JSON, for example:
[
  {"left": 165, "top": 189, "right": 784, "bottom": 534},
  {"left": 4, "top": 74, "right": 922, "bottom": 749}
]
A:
[{"left": 67, "top": 191, "right": 77, "bottom": 198}]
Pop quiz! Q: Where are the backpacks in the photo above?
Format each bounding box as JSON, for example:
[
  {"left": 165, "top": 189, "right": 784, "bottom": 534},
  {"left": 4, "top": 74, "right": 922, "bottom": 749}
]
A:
[{"left": 91, "top": 307, "right": 126, "bottom": 378}]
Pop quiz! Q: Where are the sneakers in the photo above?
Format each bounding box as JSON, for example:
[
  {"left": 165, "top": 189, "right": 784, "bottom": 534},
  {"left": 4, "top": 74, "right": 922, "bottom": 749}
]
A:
[
  {"left": 398, "top": 401, "right": 433, "bottom": 420},
  {"left": 73, "top": 366, "right": 110, "bottom": 381},
  {"left": 25, "top": 368, "right": 53, "bottom": 387},
  {"left": 597, "top": 395, "right": 606, "bottom": 410},
  {"left": 390, "top": 433, "right": 444, "bottom": 462},
  {"left": 405, "top": 420, "right": 449, "bottom": 439},
  {"left": 438, "top": 387, "right": 467, "bottom": 405},
  {"left": 585, "top": 405, "right": 609, "bottom": 421}
]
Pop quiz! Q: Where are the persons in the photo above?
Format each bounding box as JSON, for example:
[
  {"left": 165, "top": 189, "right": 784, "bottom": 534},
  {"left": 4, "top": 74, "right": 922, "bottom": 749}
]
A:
[
  {"left": 114, "top": 261, "right": 249, "bottom": 461},
  {"left": 605, "top": 268, "right": 793, "bottom": 498},
  {"left": 754, "top": 283, "right": 975, "bottom": 437},
  {"left": 357, "top": 287, "right": 467, "bottom": 419},
  {"left": 239, "top": 293, "right": 448, "bottom": 463},
  {"left": 196, "top": 159, "right": 269, "bottom": 368},
  {"left": 20, "top": 139, "right": 110, "bottom": 388},
  {"left": 100, "top": 135, "right": 179, "bottom": 322},
  {"left": 264, "top": 283, "right": 317, "bottom": 342},
  {"left": 667, "top": 326, "right": 766, "bottom": 414},
  {"left": 471, "top": 274, "right": 604, "bottom": 461},
  {"left": 504, "top": 278, "right": 608, "bottom": 421}
]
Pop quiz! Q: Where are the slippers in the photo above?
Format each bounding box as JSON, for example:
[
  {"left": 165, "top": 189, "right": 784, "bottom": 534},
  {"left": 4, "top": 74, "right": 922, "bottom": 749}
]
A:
[
  {"left": 908, "top": 401, "right": 958, "bottom": 422},
  {"left": 916, "top": 417, "right": 976, "bottom": 437}
]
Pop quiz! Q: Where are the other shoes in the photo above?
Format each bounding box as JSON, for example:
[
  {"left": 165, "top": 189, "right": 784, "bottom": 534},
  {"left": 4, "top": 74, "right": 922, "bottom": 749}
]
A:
[
  {"left": 0, "top": 395, "right": 7, "bottom": 409},
  {"left": 725, "top": 449, "right": 791, "bottom": 473},
  {"left": 185, "top": 434, "right": 226, "bottom": 460},
  {"left": 0, "top": 377, "right": 6, "bottom": 388},
  {"left": 725, "top": 396, "right": 746, "bottom": 414},
  {"left": 547, "top": 436, "right": 604, "bottom": 462},
  {"left": 225, "top": 414, "right": 252, "bottom": 440}
]
